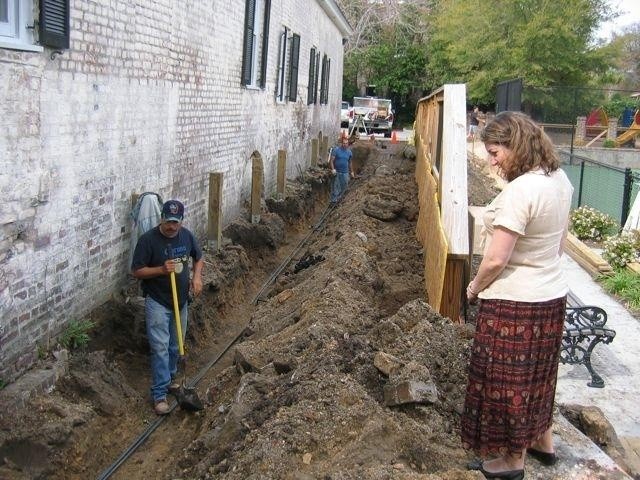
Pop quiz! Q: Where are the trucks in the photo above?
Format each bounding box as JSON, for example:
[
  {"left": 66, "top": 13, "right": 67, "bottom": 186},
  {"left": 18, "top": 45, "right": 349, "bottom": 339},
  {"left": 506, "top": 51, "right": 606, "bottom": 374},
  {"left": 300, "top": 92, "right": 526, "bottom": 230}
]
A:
[{"left": 348, "top": 97, "right": 393, "bottom": 138}]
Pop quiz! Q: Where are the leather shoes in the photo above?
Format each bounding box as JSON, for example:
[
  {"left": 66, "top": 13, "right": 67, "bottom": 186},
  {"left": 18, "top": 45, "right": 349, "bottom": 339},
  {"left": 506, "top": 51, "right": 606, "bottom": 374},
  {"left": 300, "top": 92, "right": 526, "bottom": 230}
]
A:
[
  {"left": 526, "top": 448, "right": 556, "bottom": 465},
  {"left": 466, "top": 459, "right": 525, "bottom": 480}
]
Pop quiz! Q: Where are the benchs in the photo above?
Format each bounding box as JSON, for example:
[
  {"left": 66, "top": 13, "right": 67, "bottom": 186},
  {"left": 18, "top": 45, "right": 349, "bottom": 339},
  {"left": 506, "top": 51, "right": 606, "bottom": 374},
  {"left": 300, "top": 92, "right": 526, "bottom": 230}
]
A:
[{"left": 558, "top": 289, "right": 617, "bottom": 388}]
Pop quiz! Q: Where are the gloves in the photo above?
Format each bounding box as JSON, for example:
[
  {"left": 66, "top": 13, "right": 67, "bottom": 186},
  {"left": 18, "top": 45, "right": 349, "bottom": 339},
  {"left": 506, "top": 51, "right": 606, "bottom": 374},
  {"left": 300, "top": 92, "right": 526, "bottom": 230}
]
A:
[{"left": 331, "top": 168, "right": 354, "bottom": 177}]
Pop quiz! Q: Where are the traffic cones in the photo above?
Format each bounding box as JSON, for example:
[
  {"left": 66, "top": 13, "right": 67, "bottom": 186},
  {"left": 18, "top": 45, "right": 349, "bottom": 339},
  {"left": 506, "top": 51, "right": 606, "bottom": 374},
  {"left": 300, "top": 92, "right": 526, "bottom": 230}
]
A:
[
  {"left": 343, "top": 130, "right": 347, "bottom": 142},
  {"left": 392, "top": 132, "right": 399, "bottom": 144}
]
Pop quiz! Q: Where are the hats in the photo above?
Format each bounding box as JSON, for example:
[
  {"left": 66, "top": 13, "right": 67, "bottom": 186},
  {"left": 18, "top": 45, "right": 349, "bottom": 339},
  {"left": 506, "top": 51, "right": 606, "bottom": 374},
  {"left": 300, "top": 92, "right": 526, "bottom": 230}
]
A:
[{"left": 161, "top": 200, "right": 185, "bottom": 222}]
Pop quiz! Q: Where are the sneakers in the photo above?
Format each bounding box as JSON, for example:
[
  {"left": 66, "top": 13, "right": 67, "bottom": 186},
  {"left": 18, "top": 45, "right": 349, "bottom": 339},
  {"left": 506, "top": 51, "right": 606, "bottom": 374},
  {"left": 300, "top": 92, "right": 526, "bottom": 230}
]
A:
[{"left": 153, "top": 396, "right": 171, "bottom": 415}]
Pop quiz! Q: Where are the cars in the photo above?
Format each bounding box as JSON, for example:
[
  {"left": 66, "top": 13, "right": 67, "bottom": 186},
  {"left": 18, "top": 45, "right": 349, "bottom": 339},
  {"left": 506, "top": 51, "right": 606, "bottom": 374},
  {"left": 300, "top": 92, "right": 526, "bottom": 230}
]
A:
[{"left": 341, "top": 101, "right": 352, "bottom": 129}]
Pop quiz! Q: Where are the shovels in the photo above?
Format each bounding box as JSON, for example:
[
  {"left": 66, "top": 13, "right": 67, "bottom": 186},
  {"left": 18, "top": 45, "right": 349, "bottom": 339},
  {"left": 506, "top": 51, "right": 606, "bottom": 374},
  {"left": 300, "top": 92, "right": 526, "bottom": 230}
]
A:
[{"left": 166, "top": 244, "right": 204, "bottom": 411}]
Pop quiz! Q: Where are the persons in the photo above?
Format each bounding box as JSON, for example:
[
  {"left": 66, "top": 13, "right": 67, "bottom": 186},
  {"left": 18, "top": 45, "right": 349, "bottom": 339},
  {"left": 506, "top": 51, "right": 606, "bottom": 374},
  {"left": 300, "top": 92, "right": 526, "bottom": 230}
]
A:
[
  {"left": 330, "top": 139, "right": 354, "bottom": 203},
  {"left": 131, "top": 200, "right": 203, "bottom": 415},
  {"left": 461, "top": 112, "right": 573, "bottom": 480}
]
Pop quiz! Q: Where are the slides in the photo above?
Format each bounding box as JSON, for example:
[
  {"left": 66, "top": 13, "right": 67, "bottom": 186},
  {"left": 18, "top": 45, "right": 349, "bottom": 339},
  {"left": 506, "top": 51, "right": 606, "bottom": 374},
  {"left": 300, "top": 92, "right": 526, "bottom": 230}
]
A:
[{"left": 616, "top": 109, "right": 640, "bottom": 144}]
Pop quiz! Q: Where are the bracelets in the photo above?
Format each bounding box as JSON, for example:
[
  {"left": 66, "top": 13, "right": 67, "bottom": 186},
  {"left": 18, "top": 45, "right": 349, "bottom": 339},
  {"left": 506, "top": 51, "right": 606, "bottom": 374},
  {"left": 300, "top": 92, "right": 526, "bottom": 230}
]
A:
[{"left": 468, "top": 281, "right": 477, "bottom": 296}]
